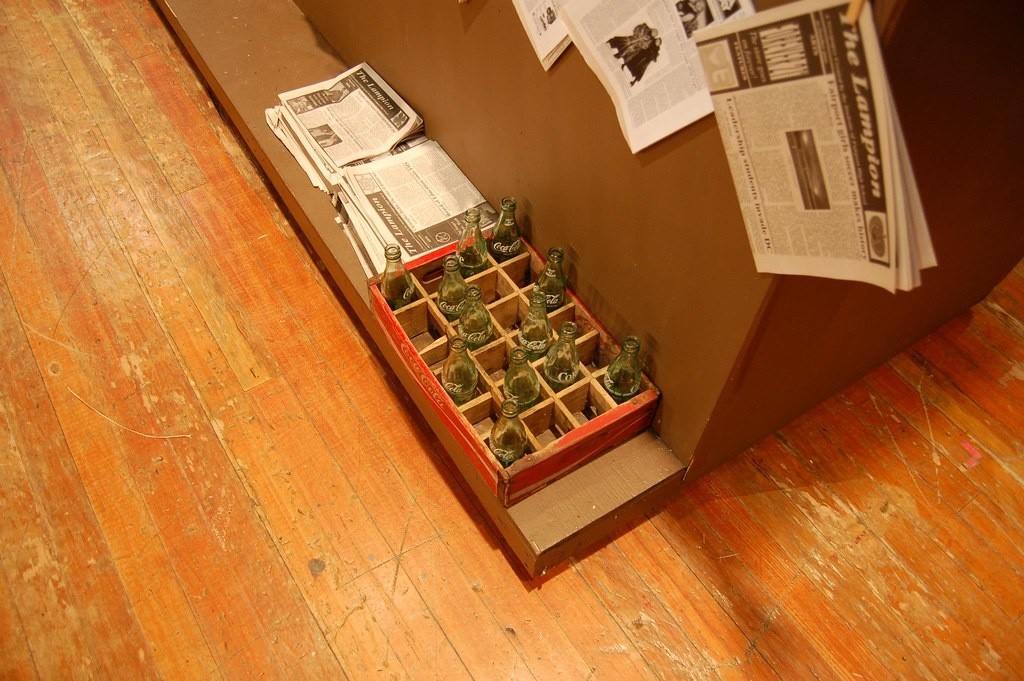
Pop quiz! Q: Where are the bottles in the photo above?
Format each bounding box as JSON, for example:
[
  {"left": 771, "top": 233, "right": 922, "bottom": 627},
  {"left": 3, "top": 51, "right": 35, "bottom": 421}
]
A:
[
  {"left": 381, "top": 243, "right": 417, "bottom": 312},
  {"left": 456, "top": 284, "right": 494, "bottom": 352},
  {"left": 518, "top": 291, "right": 553, "bottom": 364},
  {"left": 437, "top": 255, "right": 469, "bottom": 323},
  {"left": 536, "top": 246, "right": 566, "bottom": 314},
  {"left": 541, "top": 320, "right": 581, "bottom": 394},
  {"left": 456, "top": 205, "right": 490, "bottom": 279},
  {"left": 441, "top": 335, "right": 479, "bottom": 407},
  {"left": 490, "top": 194, "right": 523, "bottom": 264},
  {"left": 603, "top": 336, "right": 643, "bottom": 405},
  {"left": 503, "top": 346, "right": 541, "bottom": 415},
  {"left": 489, "top": 399, "right": 528, "bottom": 469}
]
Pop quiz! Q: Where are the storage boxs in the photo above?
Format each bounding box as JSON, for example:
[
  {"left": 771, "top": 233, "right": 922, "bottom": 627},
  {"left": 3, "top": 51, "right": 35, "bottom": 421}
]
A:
[{"left": 367, "top": 232, "right": 663, "bottom": 508}]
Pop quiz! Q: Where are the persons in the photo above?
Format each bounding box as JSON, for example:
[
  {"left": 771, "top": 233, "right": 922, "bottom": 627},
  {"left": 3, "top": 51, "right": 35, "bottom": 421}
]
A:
[
  {"left": 675, "top": 0, "right": 706, "bottom": 23},
  {"left": 478, "top": 207, "right": 498, "bottom": 224},
  {"left": 327, "top": 87, "right": 346, "bottom": 102},
  {"left": 607, "top": 23, "right": 662, "bottom": 86},
  {"left": 288, "top": 100, "right": 309, "bottom": 113},
  {"left": 309, "top": 127, "right": 339, "bottom": 147}
]
